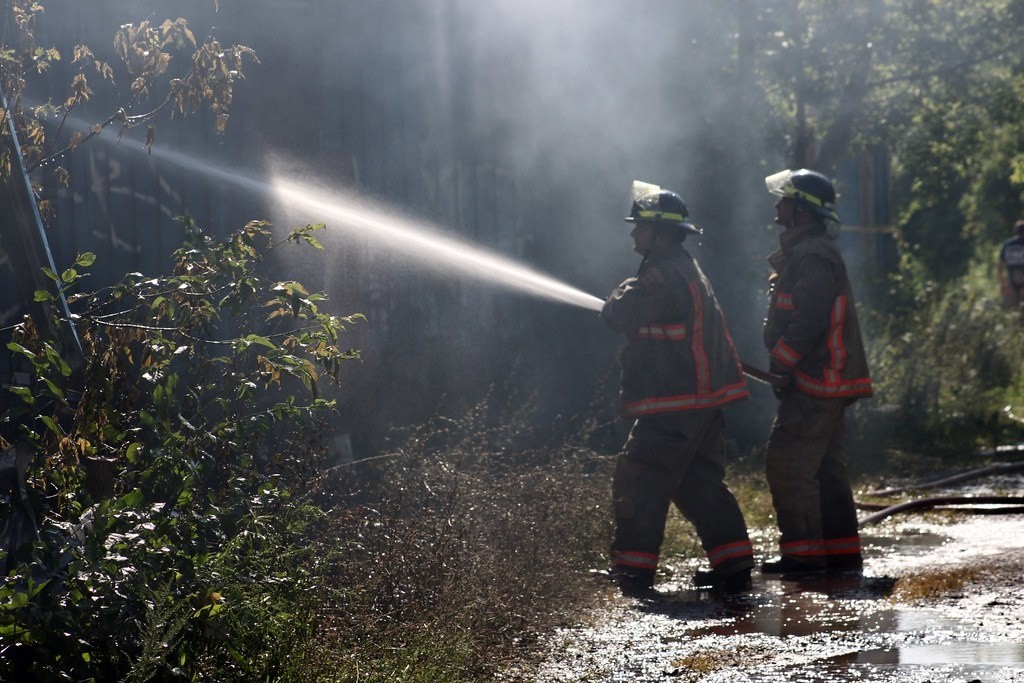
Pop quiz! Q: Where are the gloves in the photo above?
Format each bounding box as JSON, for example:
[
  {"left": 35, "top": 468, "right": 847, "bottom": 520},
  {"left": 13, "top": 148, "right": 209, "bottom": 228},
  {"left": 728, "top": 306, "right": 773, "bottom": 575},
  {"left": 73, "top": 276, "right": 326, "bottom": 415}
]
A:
[{"left": 769, "top": 368, "right": 794, "bottom": 402}]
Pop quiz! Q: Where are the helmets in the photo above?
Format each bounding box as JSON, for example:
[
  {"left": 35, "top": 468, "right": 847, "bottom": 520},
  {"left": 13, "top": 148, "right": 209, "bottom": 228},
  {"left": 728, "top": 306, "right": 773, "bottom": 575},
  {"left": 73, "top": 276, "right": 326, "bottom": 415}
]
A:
[
  {"left": 623, "top": 179, "right": 705, "bottom": 235},
  {"left": 765, "top": 168, "right": 840, "bottom": 223}
]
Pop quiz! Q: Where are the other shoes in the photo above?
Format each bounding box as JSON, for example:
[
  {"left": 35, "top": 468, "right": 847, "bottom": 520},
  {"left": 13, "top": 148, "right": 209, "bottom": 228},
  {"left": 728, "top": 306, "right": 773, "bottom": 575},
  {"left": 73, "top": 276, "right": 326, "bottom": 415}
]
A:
[
  {"left": 761, "top": 557, "right": 863, "bottom": 580},
  {"left": 587, "top": 569, "right": 647, "bottom": 593},
  {"left": 692, "top": 567, "right": 754, "bottom": 593}
]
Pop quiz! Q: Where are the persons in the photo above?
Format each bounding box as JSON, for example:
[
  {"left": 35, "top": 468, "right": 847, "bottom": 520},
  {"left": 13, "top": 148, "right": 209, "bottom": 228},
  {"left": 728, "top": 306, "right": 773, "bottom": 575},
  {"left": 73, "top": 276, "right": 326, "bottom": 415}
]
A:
[
  {"left": 997, "top": 220, "right": 1024, "bottom": 299},
  {"left": 589, "top": 181, "right": 756, "bottom": 590},
  {"left": 762, "top": 168, "right": 874, "bottom": 572}
]
